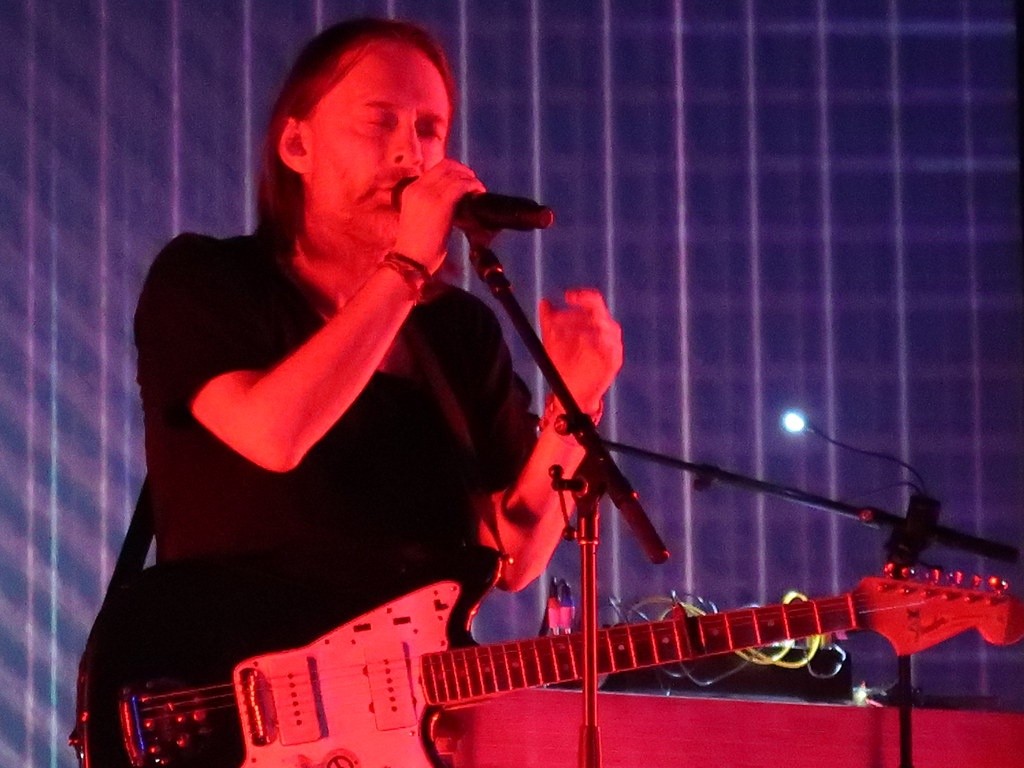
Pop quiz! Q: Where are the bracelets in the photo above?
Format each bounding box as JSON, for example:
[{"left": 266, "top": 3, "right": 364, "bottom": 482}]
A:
[
  {"left": 537, "top": 390, "right": 605, "bottom": 439},
  {"left": 379, "top": 251, "right": 441, "bottom": 305}
]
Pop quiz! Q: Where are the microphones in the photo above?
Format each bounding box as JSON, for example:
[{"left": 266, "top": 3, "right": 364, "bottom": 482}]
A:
[{"left": 392, "top": 177, "right": 553, "bottom": 234}]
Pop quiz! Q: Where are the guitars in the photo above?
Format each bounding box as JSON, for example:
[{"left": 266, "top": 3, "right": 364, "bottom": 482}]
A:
[{"left": 67, "top": 546, "right": 1023, "bottom": 768}]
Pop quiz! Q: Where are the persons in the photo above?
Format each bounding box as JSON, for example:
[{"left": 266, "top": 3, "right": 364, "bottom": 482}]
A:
[{"left": 131, "top": 18, "right": 623, "bottom": 595}]
